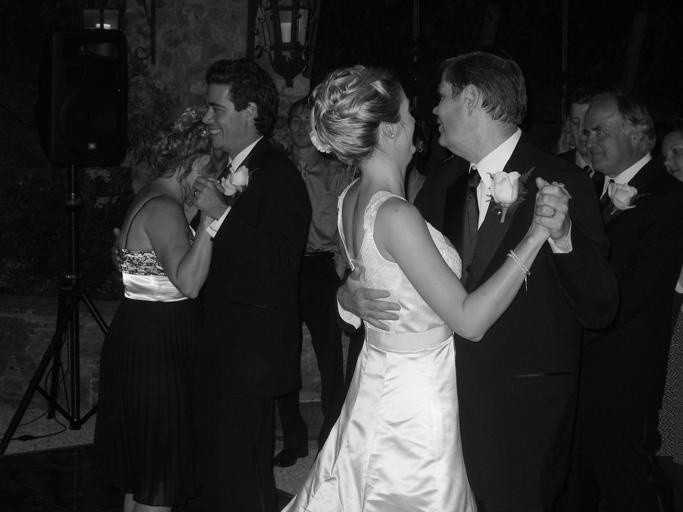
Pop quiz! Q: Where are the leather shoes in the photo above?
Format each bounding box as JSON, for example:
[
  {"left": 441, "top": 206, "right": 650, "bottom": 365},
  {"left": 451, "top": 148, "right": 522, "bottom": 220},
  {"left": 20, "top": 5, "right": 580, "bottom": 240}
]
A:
[{"left": 274, "top": 438, "right": 309, "bottom": 467}]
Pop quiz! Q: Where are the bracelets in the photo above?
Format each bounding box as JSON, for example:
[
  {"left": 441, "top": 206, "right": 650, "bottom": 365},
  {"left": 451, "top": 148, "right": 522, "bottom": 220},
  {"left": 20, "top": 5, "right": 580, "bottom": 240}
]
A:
[{"left": 505, "top": 249, "right": 532, "bottom": 292}]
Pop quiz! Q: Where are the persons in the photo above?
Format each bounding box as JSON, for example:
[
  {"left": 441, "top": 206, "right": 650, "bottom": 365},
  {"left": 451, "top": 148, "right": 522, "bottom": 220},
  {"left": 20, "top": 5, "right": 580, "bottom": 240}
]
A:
[
  {"left": 279, "top": 65, "right": 568, "bottom": 510},
  {"left": 569, "top": 93, "right": 682, "bottom": 508},
  {"left": 272, "top": 101, "right": 352, "bottom": 470},
  {"left": 658, "top": 130, "right": 681, "bottom": 177},
  {"left": 336, "top": 50, "right": 612, "bottom": 510},
  {"left": 92, "top": 107, "right": 224, "bottom": 510},
  {"left": 183, "top": 60, "right": 313, "bottom": 511},
  {"left": 529, "top": 99, "right": 596, "bottom": 179}
]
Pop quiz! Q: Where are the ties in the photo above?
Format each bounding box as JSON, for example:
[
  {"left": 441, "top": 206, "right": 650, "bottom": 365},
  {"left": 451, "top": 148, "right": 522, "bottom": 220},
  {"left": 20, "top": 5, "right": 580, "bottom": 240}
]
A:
[
  {"left": 187, "top": 166, "right": 236, "bottom": 232},
  {"left": 460, "top": 166, "right": 481, "bottom": 284}
]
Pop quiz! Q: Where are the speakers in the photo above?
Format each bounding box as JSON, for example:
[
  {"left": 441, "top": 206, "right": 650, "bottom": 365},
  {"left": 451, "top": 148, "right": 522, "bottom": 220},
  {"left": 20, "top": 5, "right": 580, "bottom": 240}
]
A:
[{"left": 38, "top": 31, "right": 128, "bottom": 166}]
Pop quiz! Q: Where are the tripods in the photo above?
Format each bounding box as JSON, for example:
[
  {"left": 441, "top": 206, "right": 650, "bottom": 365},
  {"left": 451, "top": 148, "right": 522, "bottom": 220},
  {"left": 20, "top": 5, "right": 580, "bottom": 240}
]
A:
[{"left": 1, "top": 169, "right": 109, "bottom": 463}]
